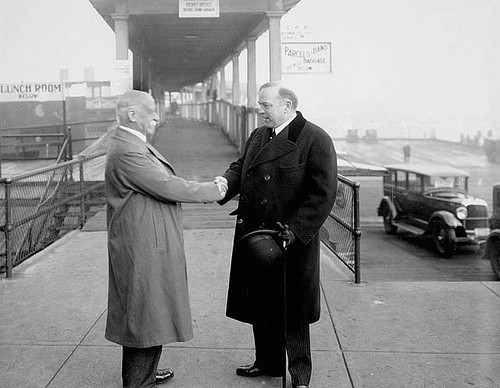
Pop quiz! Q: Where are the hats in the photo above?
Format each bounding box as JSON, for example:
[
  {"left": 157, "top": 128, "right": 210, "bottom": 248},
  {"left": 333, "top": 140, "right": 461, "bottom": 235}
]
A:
[{"left": 240, "top": 230, "right": 283, "bottom": 269}]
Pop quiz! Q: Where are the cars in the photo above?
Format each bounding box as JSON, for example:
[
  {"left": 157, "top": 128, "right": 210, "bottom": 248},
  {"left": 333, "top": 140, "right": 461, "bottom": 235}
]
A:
[{"left": 378, "top": 162, "right": 496, "bottom": 259}]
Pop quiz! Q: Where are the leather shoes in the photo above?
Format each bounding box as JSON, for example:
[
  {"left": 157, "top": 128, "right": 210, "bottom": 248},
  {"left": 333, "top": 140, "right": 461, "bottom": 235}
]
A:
[
  {"left": 236, "top": 362, "right": 282, "bottom": 377},
  {"left": 156, "top": 369, "right": 174, "bottom": 383}
]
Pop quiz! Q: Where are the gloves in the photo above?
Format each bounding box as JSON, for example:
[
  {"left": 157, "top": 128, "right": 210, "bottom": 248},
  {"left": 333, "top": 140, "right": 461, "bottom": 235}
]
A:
[{"left": 276, "top": 222, "right": 295, "bottom": 249}]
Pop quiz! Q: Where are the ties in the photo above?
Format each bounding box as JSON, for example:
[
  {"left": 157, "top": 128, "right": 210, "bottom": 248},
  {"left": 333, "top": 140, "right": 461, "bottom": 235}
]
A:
[{"left": 269, "top": 129, "right": 275, "bottom": 141}]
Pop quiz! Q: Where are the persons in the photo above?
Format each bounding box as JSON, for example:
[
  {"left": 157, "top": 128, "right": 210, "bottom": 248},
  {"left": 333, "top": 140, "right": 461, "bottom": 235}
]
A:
[
  {"left": 214, "top": 81, "right": 338, "bottom": 388},
  {"left": 103, "top": 89, "right": 228, "bottom": 388}
]
